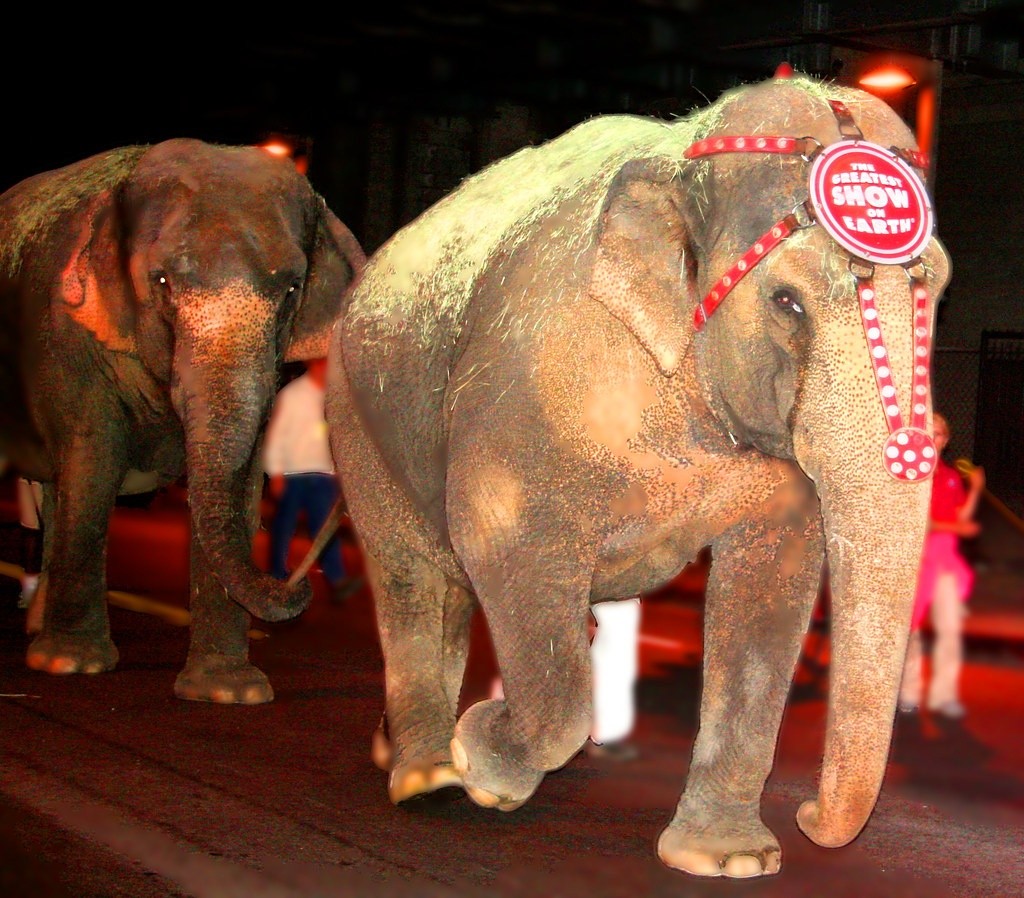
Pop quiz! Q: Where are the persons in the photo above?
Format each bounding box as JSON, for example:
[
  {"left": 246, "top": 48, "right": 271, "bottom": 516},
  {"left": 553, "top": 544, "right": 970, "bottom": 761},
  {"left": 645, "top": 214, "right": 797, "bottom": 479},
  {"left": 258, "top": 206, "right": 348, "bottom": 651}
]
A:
[
  {"left": 895, "top": 411, "right": 986, "bottom": 731},
  {"left": 259, "top": 355, "right": 355, "bottom": 609}
]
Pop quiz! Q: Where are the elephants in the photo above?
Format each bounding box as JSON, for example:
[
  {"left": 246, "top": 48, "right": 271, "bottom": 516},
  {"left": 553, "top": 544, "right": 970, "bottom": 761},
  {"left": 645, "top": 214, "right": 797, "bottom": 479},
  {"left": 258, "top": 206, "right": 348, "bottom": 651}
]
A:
[
  {"left": 287, "top": 77, "right": 952, "bottom": 879},
  {"left": 2, "top": 137, "right": 368, "bottom": 704}
]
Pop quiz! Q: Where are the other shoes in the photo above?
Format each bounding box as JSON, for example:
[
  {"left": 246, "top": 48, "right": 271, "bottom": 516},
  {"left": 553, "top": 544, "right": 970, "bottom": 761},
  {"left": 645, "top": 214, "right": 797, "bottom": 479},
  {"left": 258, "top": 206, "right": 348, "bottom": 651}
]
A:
[
  {"left": 939, "top": 700, "right": 962, "bottom": 718},
  {"left": 583, "top": 740, "right": 637, "bottom": 763},
  {"left": 898, "top": 703, "right": 919, "bottom": 715},
  {"left": 331, "top": 579, "right": 357, "bottom": 605}
]
